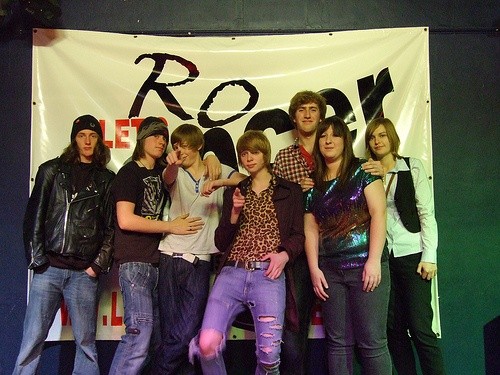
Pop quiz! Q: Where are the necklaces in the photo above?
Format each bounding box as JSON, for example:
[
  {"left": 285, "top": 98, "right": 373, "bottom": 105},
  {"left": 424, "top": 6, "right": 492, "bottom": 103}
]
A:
[{"left": 139, "top": 160, "right": 151, "bottom": 170}]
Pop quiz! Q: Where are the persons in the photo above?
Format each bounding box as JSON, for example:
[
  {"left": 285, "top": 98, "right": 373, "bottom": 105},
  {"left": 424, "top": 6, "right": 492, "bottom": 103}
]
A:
[
  {"left": 366, "top": 119, "right": 446, "bottom": 375},
  {"left": 301, "top": 115, "right": 394, "bottom": 375},
  {"left": 114, "top": 116, "right": 222, "bottom": 375},
  {"left": 6, "top": 115, "right": 118, "bottom": 375},
  {"left": 146, "top": 124, "right": 250, "bottom": 375},
  {"left": 188, "top": 130, "right": 311, "bottom": 375},
  {"left": 271, "top": 92, "right": 386, "bottom": 375}
]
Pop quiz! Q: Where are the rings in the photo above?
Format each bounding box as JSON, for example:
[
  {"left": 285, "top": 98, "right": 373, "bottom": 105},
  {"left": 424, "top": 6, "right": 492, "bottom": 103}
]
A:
[{"left": 189, "top": 227, "right": 192, "bottom": 231}]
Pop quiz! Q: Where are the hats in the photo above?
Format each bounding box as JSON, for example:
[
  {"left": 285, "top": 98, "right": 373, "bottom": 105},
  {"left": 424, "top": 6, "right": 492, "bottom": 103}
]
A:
[
  {"left": 137, "top": 117, "right": 170, "bottom": 144},
  {"left": 70, "top": 114, "right": 103, "bottom": 142}
]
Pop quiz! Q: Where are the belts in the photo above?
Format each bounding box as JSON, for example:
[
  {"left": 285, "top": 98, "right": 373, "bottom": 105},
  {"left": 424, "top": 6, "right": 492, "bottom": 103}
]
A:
[
  {"left": 222, "top": 260, "right": 270, "bottom": 271},
  {"left": 159, "top": 251, "right": 211, "bottom": 265}
]
